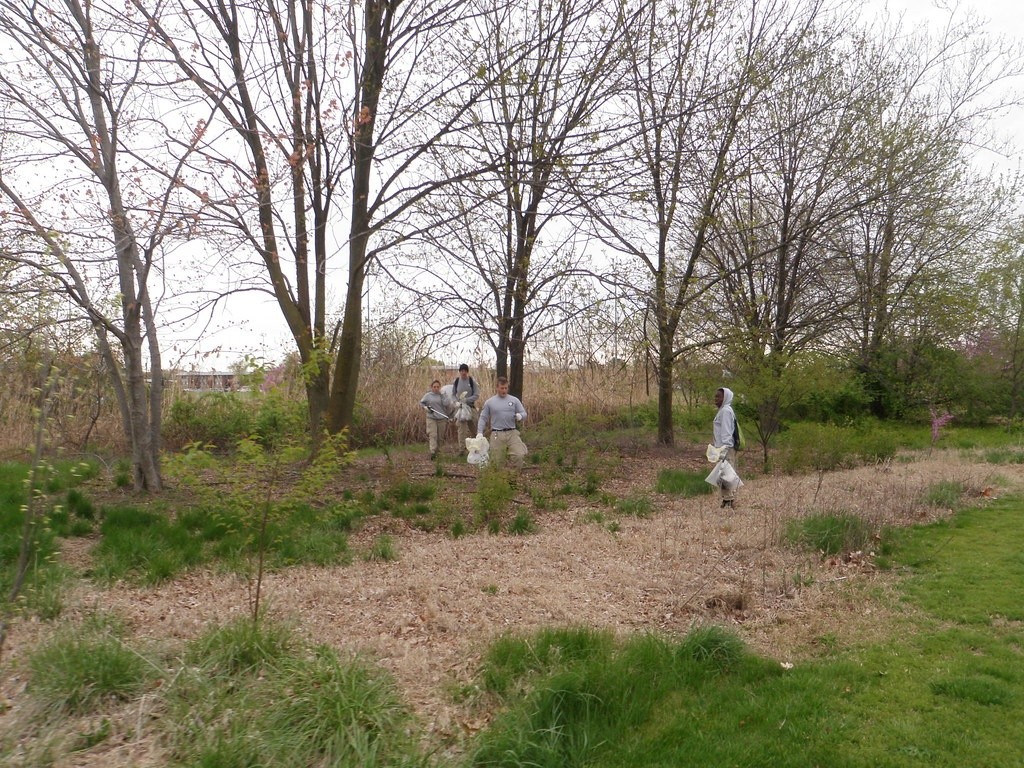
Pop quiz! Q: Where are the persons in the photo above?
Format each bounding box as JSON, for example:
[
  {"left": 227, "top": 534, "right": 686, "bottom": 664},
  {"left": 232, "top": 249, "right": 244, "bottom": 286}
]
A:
[
  {"left": 419, "top": 381, "right": 448, "bottom": 461},
  {"left": 476, "top": 378, "right": 527, "bottom": 474},
  {"left": 451, "top": 364, "right": 479, "bottom": 458},
  {"left": 705, "top": 387, "right": 746, "bottom": 509}
]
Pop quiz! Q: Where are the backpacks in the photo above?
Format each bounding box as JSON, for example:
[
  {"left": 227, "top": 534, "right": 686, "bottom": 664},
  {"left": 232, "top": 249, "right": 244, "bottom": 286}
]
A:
[{"left": 732, "top": 417, "right": 745, "bottom": 450}]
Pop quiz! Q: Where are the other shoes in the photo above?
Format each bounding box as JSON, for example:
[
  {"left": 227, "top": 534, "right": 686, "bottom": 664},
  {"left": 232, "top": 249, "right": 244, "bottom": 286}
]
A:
[
  {"left": 720, "top": 501, "right": 732, "bottom": 508},
  {"left": 430, "top": 454, "right": 435, "bottom": 460}
]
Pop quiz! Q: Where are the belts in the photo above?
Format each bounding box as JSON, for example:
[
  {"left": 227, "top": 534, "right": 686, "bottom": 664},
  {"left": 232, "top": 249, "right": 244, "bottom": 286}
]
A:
[{"left": 492, "top": 428, "right": 516, "bottom": 431}]
